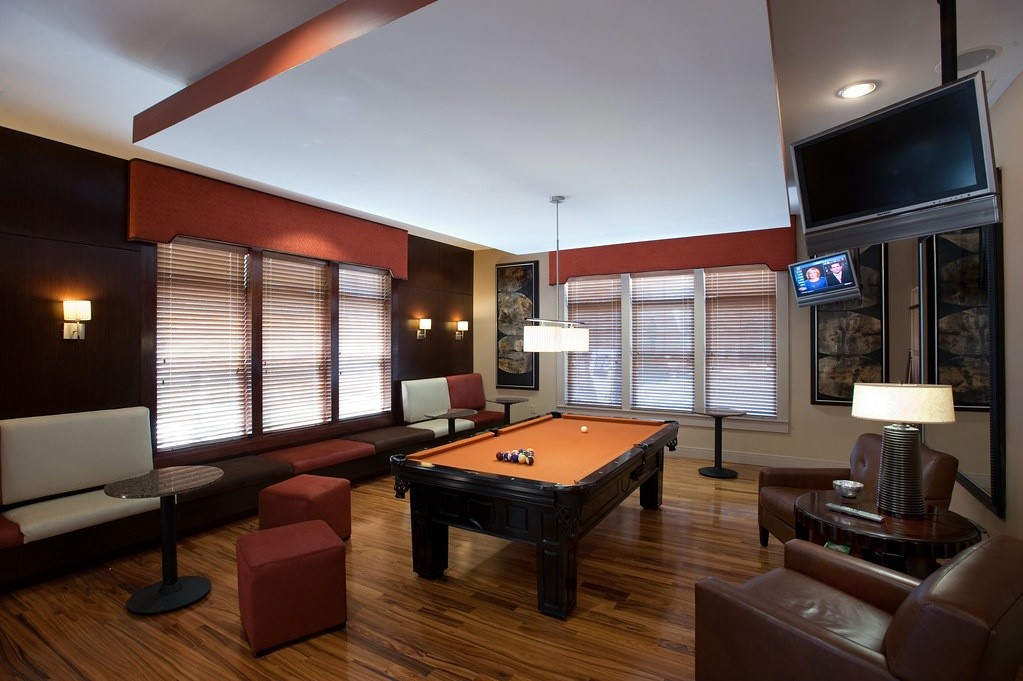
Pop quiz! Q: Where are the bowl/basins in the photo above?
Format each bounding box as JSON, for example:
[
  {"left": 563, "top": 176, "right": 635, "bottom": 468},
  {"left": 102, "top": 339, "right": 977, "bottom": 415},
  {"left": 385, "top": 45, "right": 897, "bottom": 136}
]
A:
[{"left": 832, "top": 480, "right": 864, "bottom": 497}]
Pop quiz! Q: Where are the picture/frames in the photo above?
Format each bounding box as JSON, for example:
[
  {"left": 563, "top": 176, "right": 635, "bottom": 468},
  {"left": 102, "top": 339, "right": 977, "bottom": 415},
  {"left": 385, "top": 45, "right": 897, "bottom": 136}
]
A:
[
  {"left": 927, "top": 228, "right": 989, "bottom": 412},
  {"left": 495, "top": 260, "right": 540, "bottom": 390},
  {"left": 811, "top": 241, "right": 890, "bottom": 406}
]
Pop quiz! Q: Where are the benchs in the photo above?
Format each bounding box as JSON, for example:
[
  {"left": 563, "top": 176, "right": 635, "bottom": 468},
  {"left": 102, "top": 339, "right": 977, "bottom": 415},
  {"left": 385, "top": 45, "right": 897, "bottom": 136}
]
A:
[{"left": 0, "top": 373, "right": 504, "bottom": 599}]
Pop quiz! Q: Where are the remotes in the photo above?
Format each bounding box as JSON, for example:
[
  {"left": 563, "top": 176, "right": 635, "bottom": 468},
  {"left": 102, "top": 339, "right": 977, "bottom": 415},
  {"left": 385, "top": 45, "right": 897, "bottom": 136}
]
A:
[{"left": 826, "top": 502, "right": 884, "bottom": 523}]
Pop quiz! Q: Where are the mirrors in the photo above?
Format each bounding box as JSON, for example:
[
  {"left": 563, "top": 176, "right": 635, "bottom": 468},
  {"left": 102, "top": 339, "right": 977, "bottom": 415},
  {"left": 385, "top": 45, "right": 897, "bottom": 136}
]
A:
[{"left": 917, "top": 222, "right": 1006, "bottom": 521}]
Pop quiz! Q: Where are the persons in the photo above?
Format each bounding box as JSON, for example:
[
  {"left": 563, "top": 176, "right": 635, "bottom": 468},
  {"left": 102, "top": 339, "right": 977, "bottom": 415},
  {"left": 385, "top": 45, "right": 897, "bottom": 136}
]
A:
[
  {"left": 826, "top": 262, "right": 852, "bottom": 286},
  {"left": 798, "top": 266, "right": 829, "bottom": 295}
]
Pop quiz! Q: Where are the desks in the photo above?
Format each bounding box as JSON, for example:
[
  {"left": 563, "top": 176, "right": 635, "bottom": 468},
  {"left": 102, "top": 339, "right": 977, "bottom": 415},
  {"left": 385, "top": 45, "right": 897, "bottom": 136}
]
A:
[
  {"left": 693, "top": 408, "right": 747, "bottom": 477},
  {"left": 105, "top": 466, "right": 224, "bottom": 615},
  {"left": 390, "top": 411, "right": 679, "bottom": 619},
  {"left": 424, "top": 408, "right": 478, "bottom": 440},
  {"left": 795, "top": 490, "right": 982, "bottom": 579},
  {"left": 486, "top": 397, "right": 529, "bottom": 426}
]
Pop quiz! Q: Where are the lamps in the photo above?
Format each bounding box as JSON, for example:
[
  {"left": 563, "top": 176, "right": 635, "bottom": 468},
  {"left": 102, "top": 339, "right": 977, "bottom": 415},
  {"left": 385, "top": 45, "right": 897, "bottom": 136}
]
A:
[
  {"left": 456, "top": 321, "right": 468, "bottom": 340},
  {"left": 852, "top": 383, "right": 956, "bottom": 519},
  {"left": 416, "top": 319, "right": 432, "bottom": 340},
  {"left": 523, "top": 195, "right": 590, "bottom": 352},
  {"left": 63, "top": 300, "right": 92, "bottom": 349}
]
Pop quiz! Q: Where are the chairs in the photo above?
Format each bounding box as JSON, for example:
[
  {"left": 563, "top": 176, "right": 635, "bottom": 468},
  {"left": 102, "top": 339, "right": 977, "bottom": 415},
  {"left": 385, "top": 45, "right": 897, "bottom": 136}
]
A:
[
  {"left": 758, "top": 433, "right": 960, "bottom": 544},
  {"left": 696, "top": 532, "right": 1023, "bottom": 681}
]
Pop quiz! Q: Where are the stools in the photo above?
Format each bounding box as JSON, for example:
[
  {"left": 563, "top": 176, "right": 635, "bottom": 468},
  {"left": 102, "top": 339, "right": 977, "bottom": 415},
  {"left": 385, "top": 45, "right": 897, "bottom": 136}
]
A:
[
  {"left": 258, "top": 474, "right": 351, "bottom": 542},
  {"left": 236, "top": 520, "right": 349, "bottom": 657}
]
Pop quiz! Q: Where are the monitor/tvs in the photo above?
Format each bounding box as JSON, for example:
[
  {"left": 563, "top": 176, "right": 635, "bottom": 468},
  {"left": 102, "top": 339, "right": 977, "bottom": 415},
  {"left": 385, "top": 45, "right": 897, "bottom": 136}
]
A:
[
  {"left": 788, "top": 70, "right": 1002, "bottom": 258},
  {"left": 788, "top": 249, "right": 861, "bottom": 307}
]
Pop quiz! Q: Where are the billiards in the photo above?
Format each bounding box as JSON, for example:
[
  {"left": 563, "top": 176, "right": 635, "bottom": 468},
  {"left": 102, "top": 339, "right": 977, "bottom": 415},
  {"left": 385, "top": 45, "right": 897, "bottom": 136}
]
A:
[
  {"left": 495, "top": 447, "right": 535, "bottom": 465},
  {"left": 581, "top": 426, "right": 588, "bottom": 432}
]
